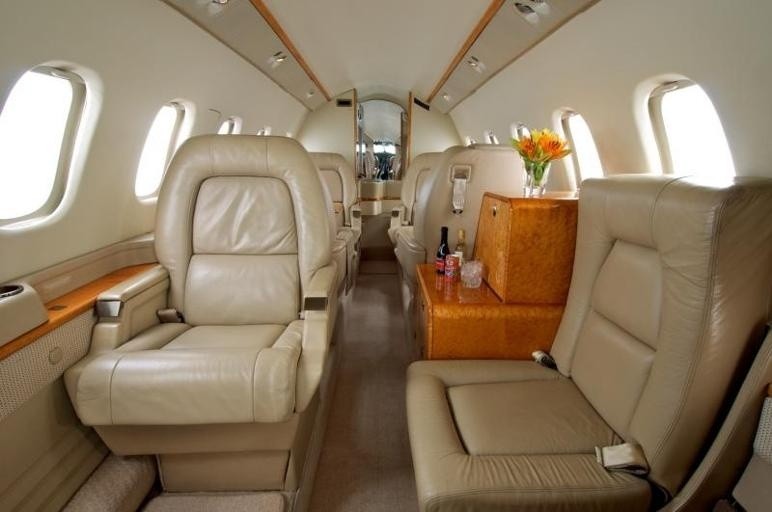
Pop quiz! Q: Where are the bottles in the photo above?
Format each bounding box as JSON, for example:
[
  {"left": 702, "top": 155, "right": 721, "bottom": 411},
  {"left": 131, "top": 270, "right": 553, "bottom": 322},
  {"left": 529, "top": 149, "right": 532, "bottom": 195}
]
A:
[
  {"left": 454, "top": 229, "right": 468, "bottom": 268},
  {"left": 434, "top": 225, "right": 450, "bottom": 274}
]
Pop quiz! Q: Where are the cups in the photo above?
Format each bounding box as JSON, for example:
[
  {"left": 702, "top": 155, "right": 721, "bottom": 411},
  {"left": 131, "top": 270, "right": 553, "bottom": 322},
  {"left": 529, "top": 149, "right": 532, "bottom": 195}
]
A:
[{"left": 459, "top": 261, "right": 484, "bottom": 290}]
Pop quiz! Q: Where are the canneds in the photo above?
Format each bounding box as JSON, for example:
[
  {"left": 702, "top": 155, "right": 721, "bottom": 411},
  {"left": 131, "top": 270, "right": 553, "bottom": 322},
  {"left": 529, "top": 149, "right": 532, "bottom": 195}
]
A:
[{"left": 445, "top": 254, "right": 460, "bottom": 284}]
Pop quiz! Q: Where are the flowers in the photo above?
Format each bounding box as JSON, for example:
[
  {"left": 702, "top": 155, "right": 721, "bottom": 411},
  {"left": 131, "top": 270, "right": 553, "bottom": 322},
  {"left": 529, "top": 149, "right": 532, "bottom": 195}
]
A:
[{"left": 510, "top": 128, "right": 574, "bottom": 187}]
